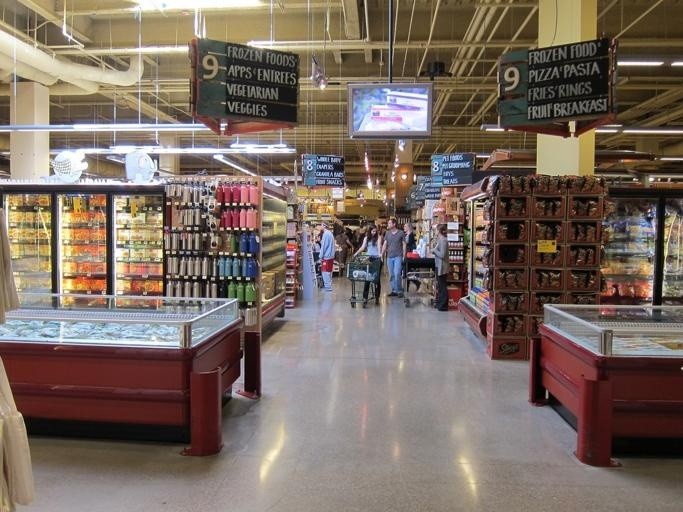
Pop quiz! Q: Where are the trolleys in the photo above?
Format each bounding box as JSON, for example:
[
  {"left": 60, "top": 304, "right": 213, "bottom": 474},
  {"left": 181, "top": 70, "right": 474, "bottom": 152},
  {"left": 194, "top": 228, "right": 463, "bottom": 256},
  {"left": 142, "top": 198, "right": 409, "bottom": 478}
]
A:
[
  {"left": 401, "top": 258, "right": 436, "bottom": 307},
  {"left": 346, "top": 252, "right": 384, "bottom": 308}
]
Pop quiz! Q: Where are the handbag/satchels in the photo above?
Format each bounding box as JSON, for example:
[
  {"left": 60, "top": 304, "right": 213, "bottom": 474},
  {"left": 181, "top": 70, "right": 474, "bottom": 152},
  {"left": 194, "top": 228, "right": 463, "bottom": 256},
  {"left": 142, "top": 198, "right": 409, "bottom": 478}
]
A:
[{"left": 321, "top": 258, "right": 334, "bottom": 272}]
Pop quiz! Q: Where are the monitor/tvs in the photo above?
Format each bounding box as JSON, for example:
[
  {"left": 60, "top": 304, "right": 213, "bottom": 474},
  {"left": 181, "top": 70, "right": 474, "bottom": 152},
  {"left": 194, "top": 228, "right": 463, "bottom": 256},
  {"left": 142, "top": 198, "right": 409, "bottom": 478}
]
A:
[{"left": 348, "top": 83, "right": 432, "bottom": 140}]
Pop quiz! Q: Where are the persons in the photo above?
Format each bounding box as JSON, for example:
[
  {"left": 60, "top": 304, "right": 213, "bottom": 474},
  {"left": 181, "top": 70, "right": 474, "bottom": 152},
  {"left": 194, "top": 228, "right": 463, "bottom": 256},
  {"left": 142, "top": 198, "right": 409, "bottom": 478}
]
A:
[
  {"left": 352, "top": 224, "right": 383, "bottom": 308},
  {"left": 380, "top": 218, "right": 407, "bottom": 298},
  {"left": 318, "top": 225, "right": 334, "bottom": 290},
  {"left": 429, "top": 224, "right": 450, "bottom": 312},
  {"left": 401, "top": 221, "right": 421, "bottom": 293},
  {"left": 325, "top": 222, "right": 384, "bottom": 274}
]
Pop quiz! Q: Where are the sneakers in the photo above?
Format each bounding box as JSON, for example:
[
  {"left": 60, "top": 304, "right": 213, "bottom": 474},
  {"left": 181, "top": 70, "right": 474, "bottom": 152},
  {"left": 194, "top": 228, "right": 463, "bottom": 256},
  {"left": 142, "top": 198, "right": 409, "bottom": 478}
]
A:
[
  {"left": 388, "top": 291, "right": 404, "bottom": 298},
  {"left": 363, "top": 298, "right": 380, "bottom": 304},
  {"left": 435, "top": 303, "right": 448, "bottom": 312}
]
